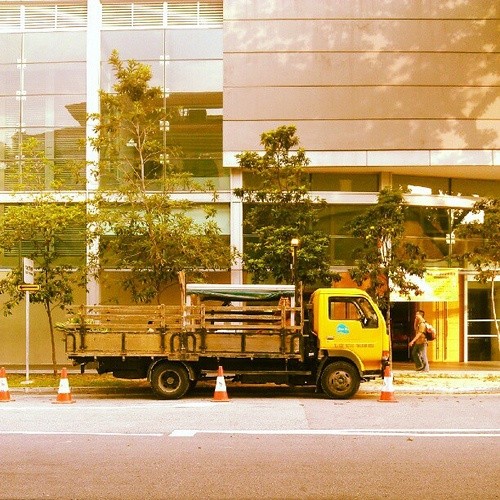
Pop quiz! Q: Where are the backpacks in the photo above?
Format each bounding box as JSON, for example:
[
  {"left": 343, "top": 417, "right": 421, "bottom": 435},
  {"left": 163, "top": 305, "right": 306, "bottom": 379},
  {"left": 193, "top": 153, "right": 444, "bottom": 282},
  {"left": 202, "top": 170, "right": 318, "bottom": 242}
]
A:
[{"left": 418, "top": 322, "right": 436, "bottom": 341}]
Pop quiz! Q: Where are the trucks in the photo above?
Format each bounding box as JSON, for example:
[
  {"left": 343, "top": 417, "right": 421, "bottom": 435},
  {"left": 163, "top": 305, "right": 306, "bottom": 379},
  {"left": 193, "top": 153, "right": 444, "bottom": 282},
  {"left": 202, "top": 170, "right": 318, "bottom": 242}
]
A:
[{"left": 58, "top": 283, "right": 389, "bottom": 401}]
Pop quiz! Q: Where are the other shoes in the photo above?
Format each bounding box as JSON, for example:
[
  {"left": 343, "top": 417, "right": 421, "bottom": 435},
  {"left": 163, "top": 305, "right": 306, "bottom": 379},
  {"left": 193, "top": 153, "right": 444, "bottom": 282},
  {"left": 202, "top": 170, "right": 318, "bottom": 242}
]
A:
[{"left": 416, "top": 366, "right": 429, "bottom": 373}]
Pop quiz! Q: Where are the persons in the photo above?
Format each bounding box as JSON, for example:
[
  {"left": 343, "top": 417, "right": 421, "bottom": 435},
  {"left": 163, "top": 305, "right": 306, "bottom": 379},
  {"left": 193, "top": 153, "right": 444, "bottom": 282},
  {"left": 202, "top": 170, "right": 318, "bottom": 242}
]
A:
[{"left": 408, "top": 310, "right": 429, "bottom": 373}]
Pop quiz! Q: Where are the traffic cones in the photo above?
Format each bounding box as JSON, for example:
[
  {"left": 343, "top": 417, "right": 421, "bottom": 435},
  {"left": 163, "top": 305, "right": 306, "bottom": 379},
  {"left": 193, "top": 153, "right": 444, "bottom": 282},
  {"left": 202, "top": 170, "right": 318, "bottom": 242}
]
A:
[
  {"left": 0, "top": 366, "right": 16, "bottom": 402},
  {"left": 51, "top": 367, "right": 78, "bottom": 403},
  {"left": 378, "top": 351, "right": 400, "bottom": 402},
  {"left": 208, "top": 366, "right": 233, "bottom": 402}
]
